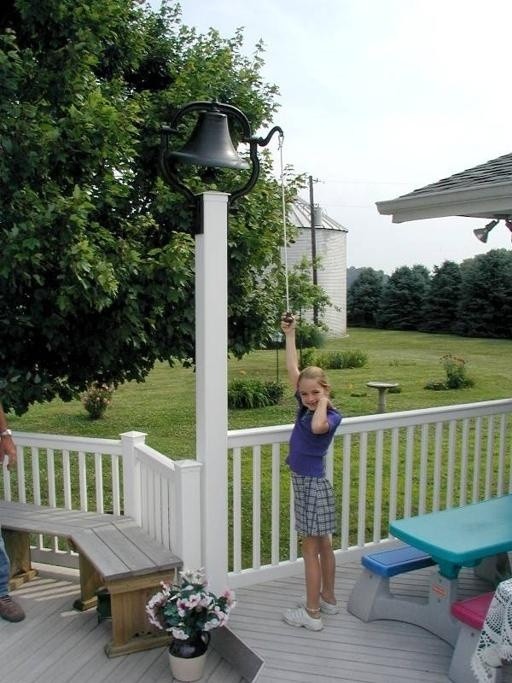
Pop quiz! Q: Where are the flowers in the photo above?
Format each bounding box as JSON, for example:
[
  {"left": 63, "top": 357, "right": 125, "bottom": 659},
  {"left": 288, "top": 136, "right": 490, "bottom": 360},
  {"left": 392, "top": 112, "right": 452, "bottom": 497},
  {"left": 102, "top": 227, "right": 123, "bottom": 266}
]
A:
[{"left": 143, "top": 563, "right": 238, "bottom": 651}]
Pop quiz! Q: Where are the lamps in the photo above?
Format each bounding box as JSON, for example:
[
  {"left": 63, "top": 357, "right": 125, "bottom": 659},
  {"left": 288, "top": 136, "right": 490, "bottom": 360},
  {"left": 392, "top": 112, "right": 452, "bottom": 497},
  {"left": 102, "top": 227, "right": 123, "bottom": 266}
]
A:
[{"left": 471, "top": 218, "right": 504, "bottom": 243}]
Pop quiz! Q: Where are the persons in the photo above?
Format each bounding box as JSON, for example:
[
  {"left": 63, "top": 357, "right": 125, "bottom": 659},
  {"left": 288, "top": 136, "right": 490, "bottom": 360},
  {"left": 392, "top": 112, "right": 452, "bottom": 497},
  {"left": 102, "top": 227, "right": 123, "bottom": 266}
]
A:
[
  {"left": 280, "top": 310, "right": 342, "bottom": 632},
  {"left": 0, "top": 406, "right": 26, "bottom": 623}
]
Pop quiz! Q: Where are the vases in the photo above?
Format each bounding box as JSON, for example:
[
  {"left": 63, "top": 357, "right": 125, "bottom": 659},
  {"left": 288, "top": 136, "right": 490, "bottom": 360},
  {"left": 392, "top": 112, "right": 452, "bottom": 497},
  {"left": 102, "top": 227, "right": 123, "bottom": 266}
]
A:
[{"left": 168, "top": 631, "right": 210, "bottom": 683}]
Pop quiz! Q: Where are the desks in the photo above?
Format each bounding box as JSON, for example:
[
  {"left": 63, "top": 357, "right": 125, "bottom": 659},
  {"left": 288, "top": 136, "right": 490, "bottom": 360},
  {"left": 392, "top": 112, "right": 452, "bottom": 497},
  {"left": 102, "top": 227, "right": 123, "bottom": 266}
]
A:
[{"left": 387, "top": 493, "right": 511, "bottom": 645}]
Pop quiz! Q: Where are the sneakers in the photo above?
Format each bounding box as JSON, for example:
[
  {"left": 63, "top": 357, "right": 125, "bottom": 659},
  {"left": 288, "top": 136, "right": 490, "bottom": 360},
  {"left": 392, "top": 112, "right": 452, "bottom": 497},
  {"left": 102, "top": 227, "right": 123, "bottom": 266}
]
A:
[
  {"left": 296, "top": 594, "right": 340, "bottom": 615},
  {"left": 1, "top": 595, "right": 25, "bottom": 622},
  {"left": 282, "top": 608, "right": 324, "bottom": 631}
]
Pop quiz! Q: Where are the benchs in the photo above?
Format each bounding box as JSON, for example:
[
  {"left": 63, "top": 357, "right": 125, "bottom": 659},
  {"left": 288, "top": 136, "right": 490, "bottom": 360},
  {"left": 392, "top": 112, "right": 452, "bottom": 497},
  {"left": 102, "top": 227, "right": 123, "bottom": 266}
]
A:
[
  {"left": 0, "top": 497, "right": 185, "bottom": 659},
  {"left": 345, "top": 546, "right": 438, "bottom": 623},
  {"left": 447, "top": 578, "right": 511, "bottom": 682}
]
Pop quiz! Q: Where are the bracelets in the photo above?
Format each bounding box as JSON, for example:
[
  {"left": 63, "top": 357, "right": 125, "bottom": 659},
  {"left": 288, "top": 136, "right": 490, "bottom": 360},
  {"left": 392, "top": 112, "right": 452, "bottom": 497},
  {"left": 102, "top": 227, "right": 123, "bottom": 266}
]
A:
[{"left": 0, "top": 428, "right": 12, "bottom": 437}]
